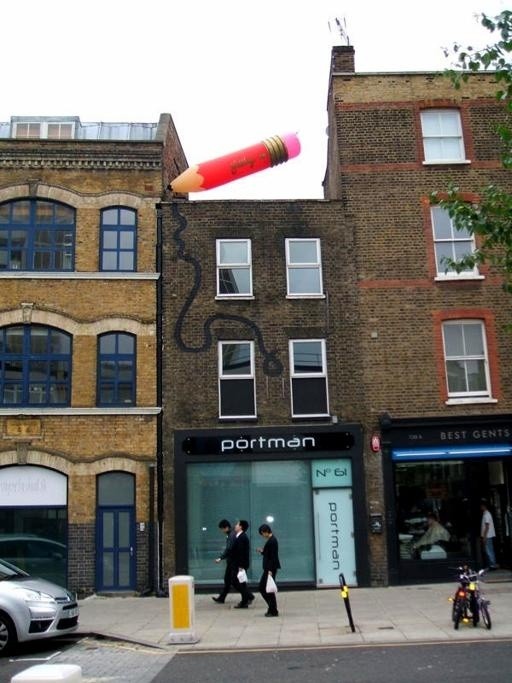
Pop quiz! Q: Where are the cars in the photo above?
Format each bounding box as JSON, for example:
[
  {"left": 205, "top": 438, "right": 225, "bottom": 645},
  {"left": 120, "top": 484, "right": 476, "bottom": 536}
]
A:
[
  {"left": 1, "top": 533, "right": 69, "bottom": 574},
  {"left": 0, "top": 556, "right": 80, "bottom": 652}
]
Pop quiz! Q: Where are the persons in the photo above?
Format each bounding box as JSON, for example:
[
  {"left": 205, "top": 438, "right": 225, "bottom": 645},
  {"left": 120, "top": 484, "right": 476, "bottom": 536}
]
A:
[
  {"left": 212, "top": 520, "right": 238, "bottom": 601},
  {"left": 412, "top": 513, "right": 451, "bottom": 560},
  {"left": 230, "top": 520, "right": 254, "bottom": 609},
  {"left": 478, "top": 501, "right": 499, "bottom": 571},
  {"left": 256, "top": 524, "right": 281, "bottom": 617}
]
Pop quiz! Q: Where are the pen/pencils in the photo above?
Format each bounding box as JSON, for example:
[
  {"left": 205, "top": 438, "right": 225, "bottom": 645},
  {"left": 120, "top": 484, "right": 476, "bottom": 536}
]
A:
[{"left": 166, "top": 132, "right": 302, "bottom": 193}]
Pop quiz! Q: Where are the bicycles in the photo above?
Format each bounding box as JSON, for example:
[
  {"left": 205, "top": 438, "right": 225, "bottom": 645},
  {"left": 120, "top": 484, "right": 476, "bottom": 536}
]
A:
[{"left": 450, "top": 565, "right": 493, "bottom": 631}]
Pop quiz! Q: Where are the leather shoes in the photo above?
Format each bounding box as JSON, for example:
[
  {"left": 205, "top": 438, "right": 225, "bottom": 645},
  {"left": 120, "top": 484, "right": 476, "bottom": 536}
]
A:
[
  {"left": 265, "top": 609, "right": 278, "bottom": 617},
  {"left": 212, "top": 596, "right": 224, "bottom": 604},
  {"left": 235, "top": 594, "right": 254, "bottom": 610}
]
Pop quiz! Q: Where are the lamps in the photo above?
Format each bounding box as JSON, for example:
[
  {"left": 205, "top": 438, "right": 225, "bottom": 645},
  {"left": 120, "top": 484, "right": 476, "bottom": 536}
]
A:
[{"left": 370, "top": 431, "right": 381, "bottom": 452}]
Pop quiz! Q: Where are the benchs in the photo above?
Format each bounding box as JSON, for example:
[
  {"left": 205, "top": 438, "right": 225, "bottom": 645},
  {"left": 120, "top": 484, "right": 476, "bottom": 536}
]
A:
[{"left": 400, "top": 556, "right": 479, "bottom": 584}]
